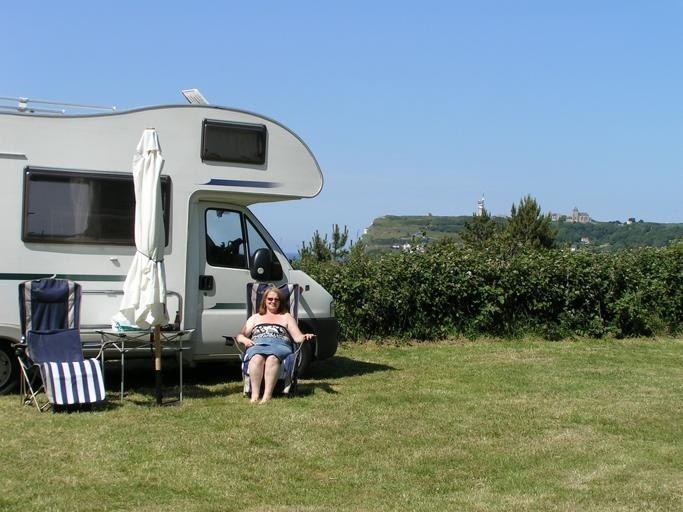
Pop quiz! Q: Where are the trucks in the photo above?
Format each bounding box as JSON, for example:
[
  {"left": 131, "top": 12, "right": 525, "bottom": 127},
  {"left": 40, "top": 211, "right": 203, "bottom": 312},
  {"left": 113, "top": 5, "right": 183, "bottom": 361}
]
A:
[{"left": 0, "top": 88, "right": 337, "bottom": 396}]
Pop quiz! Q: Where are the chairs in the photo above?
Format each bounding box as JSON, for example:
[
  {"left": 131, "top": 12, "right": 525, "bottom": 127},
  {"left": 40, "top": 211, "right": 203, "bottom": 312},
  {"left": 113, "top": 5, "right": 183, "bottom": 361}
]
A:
[
  {"left": 9, "top": 278, "right": 107, "bottom": 412},
  {"left": 223, "top": 283, "right": 318, "bottom": 398}
]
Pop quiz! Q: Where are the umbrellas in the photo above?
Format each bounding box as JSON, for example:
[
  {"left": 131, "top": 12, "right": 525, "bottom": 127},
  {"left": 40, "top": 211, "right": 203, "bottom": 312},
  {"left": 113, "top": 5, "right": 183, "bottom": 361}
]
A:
[{"left": 118, "top": 127, "right": 170, "bottom": 403}]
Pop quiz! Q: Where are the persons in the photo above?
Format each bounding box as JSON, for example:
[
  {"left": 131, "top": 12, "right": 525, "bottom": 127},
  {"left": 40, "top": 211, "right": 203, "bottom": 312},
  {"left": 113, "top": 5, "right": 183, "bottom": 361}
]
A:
[{"left": 236, "top": 285, "right": 318, "bottom": 403}]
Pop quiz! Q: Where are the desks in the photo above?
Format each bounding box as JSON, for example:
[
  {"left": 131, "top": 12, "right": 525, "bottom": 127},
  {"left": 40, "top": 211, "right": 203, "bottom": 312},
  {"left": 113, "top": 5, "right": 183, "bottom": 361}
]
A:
[{"left": 98, "top": 328, "right": 197, "bottom": 403}]
